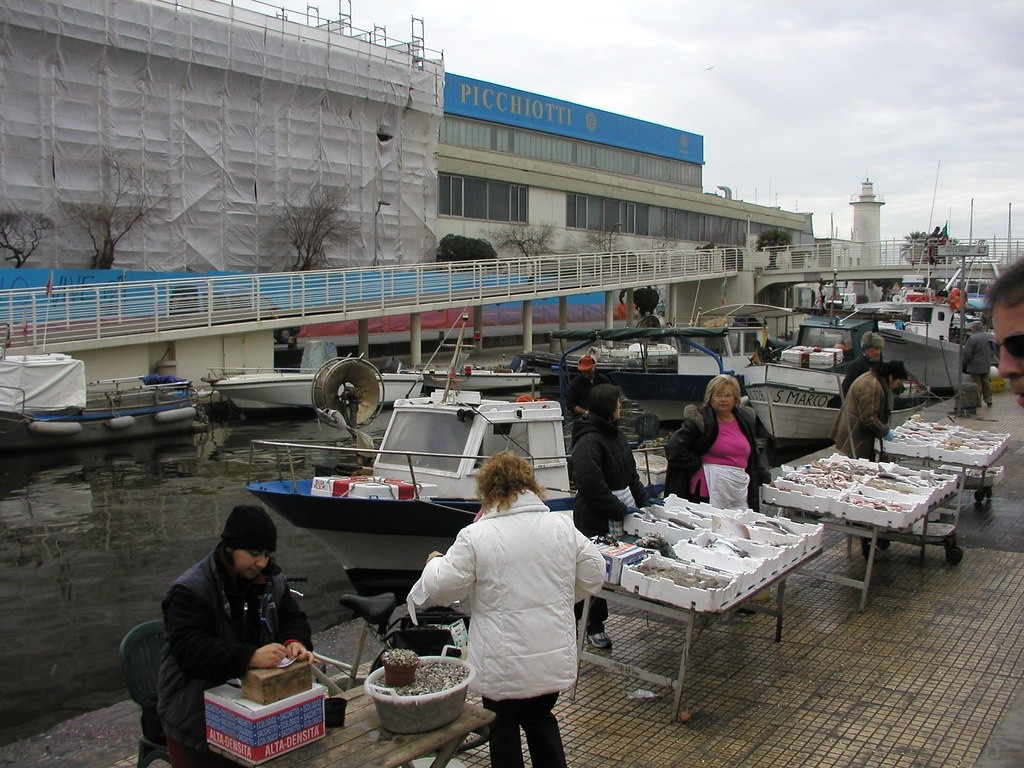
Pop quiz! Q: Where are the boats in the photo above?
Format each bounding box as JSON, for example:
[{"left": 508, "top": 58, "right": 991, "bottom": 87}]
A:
[
  {"left": 245, "top": 293, "right": 669, "bottom": 606},
  {"left": 202, "top": 160, "right": 993, "bottom": 456},
  {"left": 0, "top": 353, "right": 210, "bottom": 459}
]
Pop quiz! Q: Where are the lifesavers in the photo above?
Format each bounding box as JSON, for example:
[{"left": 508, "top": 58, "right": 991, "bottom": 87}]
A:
[{"left": 950, "top": 288, "right": 968, "bottom": 310}]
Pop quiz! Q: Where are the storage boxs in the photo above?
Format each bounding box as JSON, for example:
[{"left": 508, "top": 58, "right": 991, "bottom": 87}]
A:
[
  {"left": 791, "top": 344, "right": 817, "bottom": 353},
  {"left": 203, "top": 675, "right": 326, "bottom": 766},
  {"left": 782, "top": 349, "right": 806, "bottom": 367},
  {"left": 242, "top": 654, "right": 316, "bottom": 705},
  {"left": 309, "top": 473, "right": 439, "bottom": 498},
  {"left": 821, "top": 347, "right": 844, "bottom": 365},
  {"left": 808, "top": 352, "right": 835, "bottom": 369},
  {"left": 588, "top": 412, "right": 1010, "bottom": 615}
]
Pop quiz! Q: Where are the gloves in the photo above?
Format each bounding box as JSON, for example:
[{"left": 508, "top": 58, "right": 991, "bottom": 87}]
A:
[
  {"left": 883, "top": 431, "right": 894, "bottom": 441},
  {"left": 640, "top": 498, "right": 666, "bottom": 507},
  {"left": 628, "top": 507, "right": 645, "bottom": 514}
]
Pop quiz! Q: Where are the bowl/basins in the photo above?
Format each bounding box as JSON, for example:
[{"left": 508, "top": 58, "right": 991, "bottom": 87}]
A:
[{"left": 362, "top": 645, "right": 477, "bottom": 735}]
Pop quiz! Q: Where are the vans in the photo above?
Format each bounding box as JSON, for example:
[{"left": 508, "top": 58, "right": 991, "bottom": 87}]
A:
[{"left": 826, "top": 293, "right": 857, "bottom": 311}]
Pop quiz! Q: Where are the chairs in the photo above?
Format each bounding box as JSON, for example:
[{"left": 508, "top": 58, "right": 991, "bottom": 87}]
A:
[
  {"left": 119, "top": 618, "right": 170, "bottom": 768},
  {"left": 208, "top": 677, "right": 498, "bottom": 768}
]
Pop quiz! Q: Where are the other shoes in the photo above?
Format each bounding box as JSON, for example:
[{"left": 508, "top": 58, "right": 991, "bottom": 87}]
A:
[{"left": 988, "top": 401, "right": 992, "bottom": 407}]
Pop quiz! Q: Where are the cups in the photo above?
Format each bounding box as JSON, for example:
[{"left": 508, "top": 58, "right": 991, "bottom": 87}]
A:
[
  {"left": 324, "top": 697, "right": 347, "bottom": 728},
  {"left": 383, "top": 656, "right": 419, "bottom": 686}
]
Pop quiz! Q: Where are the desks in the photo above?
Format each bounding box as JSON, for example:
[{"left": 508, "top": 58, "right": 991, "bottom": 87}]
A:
[
  {"left": 884, "top": 445, "right": 1010, "bottom": 527},
  {"left": 566, "top": 541, "right": 829, "bottom": 723},
  {"left": 759, "top": 492, "right": 960, "bottom": 610}
]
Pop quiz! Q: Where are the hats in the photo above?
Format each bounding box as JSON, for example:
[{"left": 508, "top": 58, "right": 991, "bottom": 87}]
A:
[
  {"left": 861, "top": 331, "right": 884, "bottom": 350},
  {"left": 221, "top": 504, "right": 277, "bottom": 551},
  {"left": 578, "top": 356, "right": 595, "bottom": 371}
]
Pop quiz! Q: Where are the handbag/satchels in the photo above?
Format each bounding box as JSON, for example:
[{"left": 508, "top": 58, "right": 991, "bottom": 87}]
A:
[
  {"left": 406, "top": 575, "right": 461, "bottom": 626},
  {"left": 392, "top": 606, "right": 469, "bottom": 657}
]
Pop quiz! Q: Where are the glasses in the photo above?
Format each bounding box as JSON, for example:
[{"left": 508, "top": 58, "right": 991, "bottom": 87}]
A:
[{"left": 986, "top": 334, "right": 1024, "bottom": 361}]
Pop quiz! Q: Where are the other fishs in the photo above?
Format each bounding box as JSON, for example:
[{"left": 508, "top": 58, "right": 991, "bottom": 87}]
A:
[
  {"left": 659, "top": 538, "right": 731, "bottom": 573},
  {"left": 686, "top": 506, "right": 710, "bottom": 519},
  {"left": 702, "top": 537, "right": 752, "bottom": 559},
  {"left": 634, "top": 510, "right": 703, "bottom": 530},
  {"left": 755, "top": 520, "right": 787, "bottom": 535},
  {"left": 767, "top": 520, "right": 799, "bottom": 535},
  {"left": 710, "top": 515, "right": 751, "bottom": 540}
]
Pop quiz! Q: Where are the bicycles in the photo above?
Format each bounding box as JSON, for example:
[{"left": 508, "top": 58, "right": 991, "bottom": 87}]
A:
[{"left": 285, "top": 575, "right": 471, "bottom": 698}]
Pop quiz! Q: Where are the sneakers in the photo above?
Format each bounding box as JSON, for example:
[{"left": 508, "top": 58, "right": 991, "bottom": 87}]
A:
[{"left": 588, "top": 632, "right": 612, "bottom": 649}]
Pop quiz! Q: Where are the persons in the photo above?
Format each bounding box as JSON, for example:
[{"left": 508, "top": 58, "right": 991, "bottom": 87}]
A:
[
  {"left": 840, "top": 330, "right": 884, "bottom": 399},
  {"left": 928, "top": 226, "right": 941, "bottom": 265},
  {"left": 421, "top": 449, "right": 606, "bottom": 768},
  {"left": 827, "top": 359, "right": 909, "bottom": 559},
  {"left": 962, "top": 320, "right": 995, "bottom": 407},
  {"left": 983, "top": 256, "right": 1024, "bottom": 409},
  {"left": 566, "top": 356, "right": 631, "bottom": 449},
  {"left": 156, "top": 505, "right": 321, "bottom": 768},
  {"left": 569, "top": 384, "right": 666, "bottom": 649},
  {"left": 663, "top": 374, "right": 771, "bottom": 514}
]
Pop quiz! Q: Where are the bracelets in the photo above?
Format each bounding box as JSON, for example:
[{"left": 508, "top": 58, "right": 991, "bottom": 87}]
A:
[{"left": 582, "top": 410, "right": 589, "bottom": 417}]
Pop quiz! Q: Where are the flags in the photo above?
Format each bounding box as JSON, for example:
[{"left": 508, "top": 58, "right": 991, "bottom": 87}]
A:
[
  {"left": 720, "top": 278, "right": 727, "bottom": 307},
  {"left": 816, "top": 277, "right": 826, "bottom": 310},
  {"left": 829, "top": 278, "right": 841, "bottom": 301}
]
